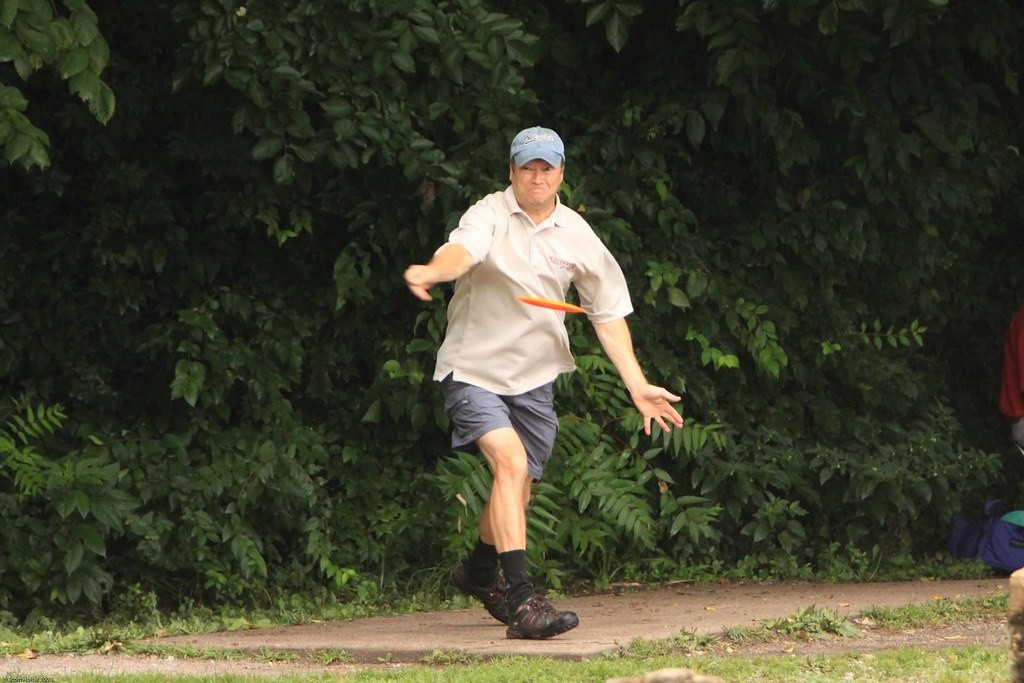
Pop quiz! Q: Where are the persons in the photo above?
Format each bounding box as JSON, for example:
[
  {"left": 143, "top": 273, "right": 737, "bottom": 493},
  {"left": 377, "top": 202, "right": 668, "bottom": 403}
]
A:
[
  {"left": 404, "top": 126, "right": 684, "bottom": 639},
  {"left": 999, "top": 304, "right": 1024, "bottom": 447}
]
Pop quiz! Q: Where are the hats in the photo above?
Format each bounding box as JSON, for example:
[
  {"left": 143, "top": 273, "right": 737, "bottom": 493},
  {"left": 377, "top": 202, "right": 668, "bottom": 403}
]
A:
[{"left": 510, "top": 125, "right": 565, "bottom": 167}]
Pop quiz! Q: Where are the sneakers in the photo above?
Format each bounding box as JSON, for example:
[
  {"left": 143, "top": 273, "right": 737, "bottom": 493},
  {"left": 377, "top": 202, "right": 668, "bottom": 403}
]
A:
[
  {"left": 507, "top": 594, "right": 580, "bottom": 638},
  {"left": 452, "top": 554, "right": 509, "bottom": 622}
]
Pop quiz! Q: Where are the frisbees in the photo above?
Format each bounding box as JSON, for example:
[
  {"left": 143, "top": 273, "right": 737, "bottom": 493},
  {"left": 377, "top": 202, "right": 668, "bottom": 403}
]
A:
[{"left": 515, "top": 296, "right": 586, "bottom": 312}]
